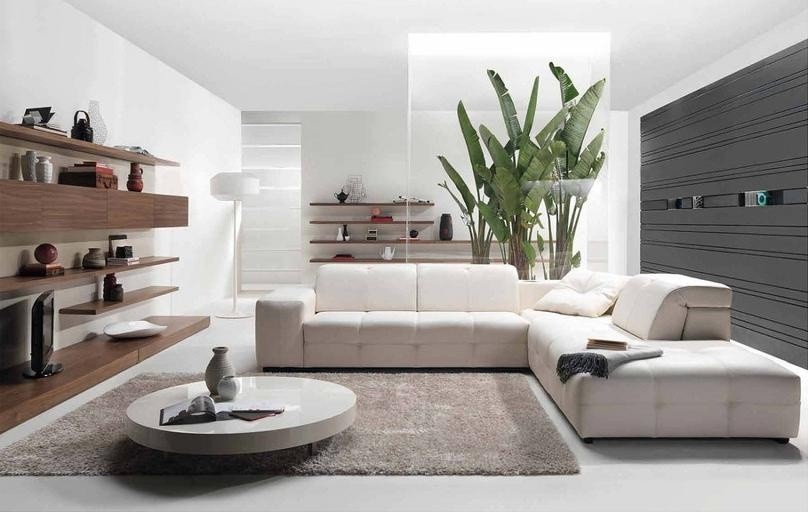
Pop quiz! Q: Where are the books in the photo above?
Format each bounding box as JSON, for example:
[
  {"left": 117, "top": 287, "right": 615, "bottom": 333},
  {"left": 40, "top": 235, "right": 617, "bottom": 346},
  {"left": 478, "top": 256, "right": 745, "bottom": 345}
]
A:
[
  {"left": 159, "top": 395, "right": 286, "bottom": 422},
  {"left": 585, "top": 337, "right": 628, "bottom": 353}
]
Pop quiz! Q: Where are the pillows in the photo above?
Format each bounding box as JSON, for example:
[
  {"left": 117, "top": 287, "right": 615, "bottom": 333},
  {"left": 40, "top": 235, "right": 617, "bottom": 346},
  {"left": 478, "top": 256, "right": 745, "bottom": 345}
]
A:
[{"left": 533, "top": 267, "right": 634, "bottom": 318}]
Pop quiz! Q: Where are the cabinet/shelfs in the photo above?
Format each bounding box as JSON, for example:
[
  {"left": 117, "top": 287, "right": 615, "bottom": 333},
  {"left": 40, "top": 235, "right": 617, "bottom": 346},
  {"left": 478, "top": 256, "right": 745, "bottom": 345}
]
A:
[
  {"left": 308, "top": 200, "right": 558, "bottom": 264},
  {"left": 1, "top": 121, "right": 212, "bottom": 435}
]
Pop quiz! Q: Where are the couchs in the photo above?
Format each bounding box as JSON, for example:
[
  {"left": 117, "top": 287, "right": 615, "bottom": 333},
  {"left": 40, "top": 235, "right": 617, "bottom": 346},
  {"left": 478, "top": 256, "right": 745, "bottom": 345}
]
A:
[
  {"left": 255, "top": 263, "right": 529, "bottom": 372},
  {"left": 529, "top": 271, "right": 802, "bottom": 444}
]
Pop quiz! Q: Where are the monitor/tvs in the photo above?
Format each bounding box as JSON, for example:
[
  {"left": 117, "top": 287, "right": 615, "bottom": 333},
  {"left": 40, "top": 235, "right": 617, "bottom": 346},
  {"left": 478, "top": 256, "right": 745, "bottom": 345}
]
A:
[{"left": 23, "top": 290, "right": 62, "bottom": 377}]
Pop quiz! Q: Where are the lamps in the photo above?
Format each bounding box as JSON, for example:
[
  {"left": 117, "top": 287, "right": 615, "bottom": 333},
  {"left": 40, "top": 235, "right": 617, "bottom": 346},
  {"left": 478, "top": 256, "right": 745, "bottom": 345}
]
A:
[{"left": 212, "top": 173, "right": 262, "bottom": 319}]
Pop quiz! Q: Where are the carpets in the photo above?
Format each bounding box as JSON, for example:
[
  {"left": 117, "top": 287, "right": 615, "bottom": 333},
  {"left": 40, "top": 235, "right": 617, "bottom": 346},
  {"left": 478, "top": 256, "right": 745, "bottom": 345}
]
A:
[{"left": 0, "top": 364, "right": 583, "bottom": 477}]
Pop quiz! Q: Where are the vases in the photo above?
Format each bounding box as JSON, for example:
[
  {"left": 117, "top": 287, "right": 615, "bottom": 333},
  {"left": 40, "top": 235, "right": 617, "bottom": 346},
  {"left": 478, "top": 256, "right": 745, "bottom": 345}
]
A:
[
  {"left": 111, "top": 283, "right": 124, "bottom": 301},
  {"left": 83, "top": 247, "right": 106, "bottom": 270},
  {"left": 21, "top": 150, "right": 40, "bottom": 182},
  {"left": 34, "top": 155, "right": 53, "bottom": 184},
  {"left": 439, "top": 214, "right": 454, "bottom": 240},
  {"left": 8, "top": 153, "right": 21, "bottom": 180},
  {"left": 127, "top": 162, "right": 144, "bottom": 192},
  {"left": 216, "top": 375, "right": 241, "bottom": 400},
  {"left": 87, "top": 99, "right": 109, "bottom": 146},
  {"left": 205, "top": 346, "right": 235, "bottom": 400},
  {"left": 102, "top": 272, "right": 116, "bottom": 301}
]
